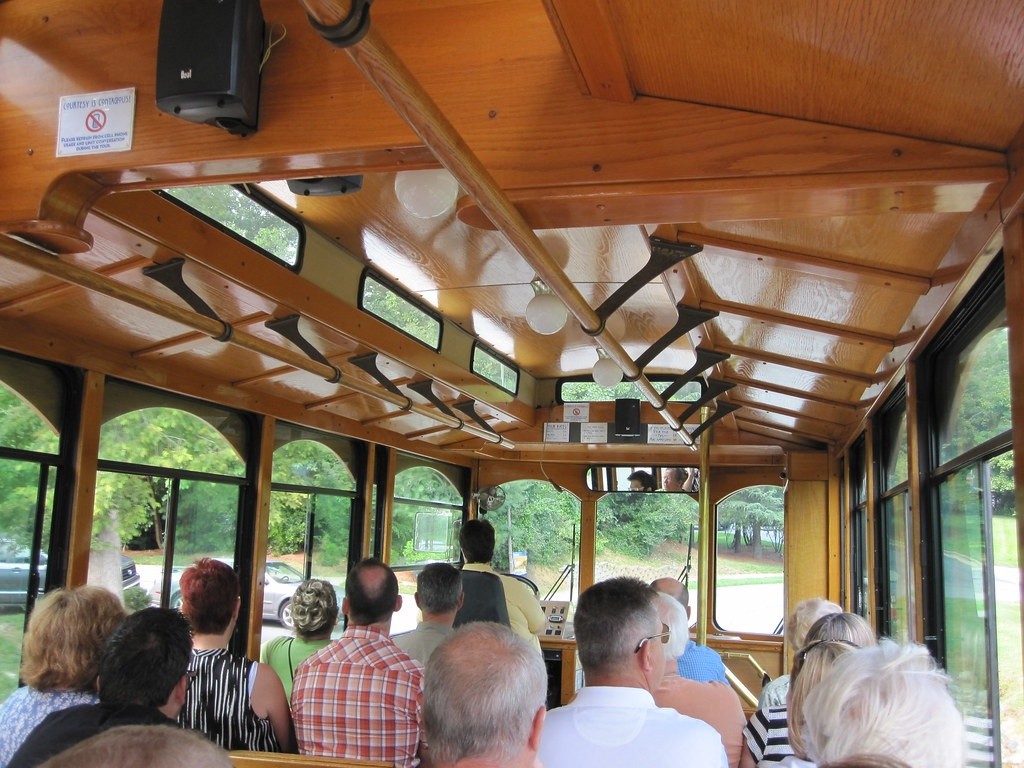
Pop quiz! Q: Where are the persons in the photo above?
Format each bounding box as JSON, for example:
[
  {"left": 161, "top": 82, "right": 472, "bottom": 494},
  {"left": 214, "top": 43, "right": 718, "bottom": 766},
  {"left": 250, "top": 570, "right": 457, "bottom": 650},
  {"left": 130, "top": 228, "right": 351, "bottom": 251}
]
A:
[
  {"left": 740, "top": 598, "right": 963, "bottom": 768},
  {"left": 259, "top": 579, "right": 340, "bottom": 708},
  {"left": 536, "top": 576, "right": 746, "bottom": 768},
  {"left": 291, "top": 558, "right": 428, "bottom": 768},
  {"left": 0, "top": 585, "right": 232, "bottom": 768},
  {"left": 628, "top": 471, "right": 656, "bottom": 491},
  {"left": 174, "top": 560, "right": 297, "bottom": 754},
  {"left": 422, "top": 622, "right": 549, "bottom": 768},
  {"left": 662, "top": 468, "right": 700, "bottom": 491},
  {"left": 459, "top": 520, "right": 547, "bottom": 656},
  {"left": 391, "top": 563, "right": 464, "bottom": 666}
]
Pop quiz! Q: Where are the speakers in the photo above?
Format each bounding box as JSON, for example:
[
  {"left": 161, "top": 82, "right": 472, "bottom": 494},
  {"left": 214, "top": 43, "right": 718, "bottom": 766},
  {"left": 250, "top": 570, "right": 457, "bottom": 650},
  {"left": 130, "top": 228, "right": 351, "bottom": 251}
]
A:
[
  {"left": 155, "top": 0, "right": 267, "bottom": 135},
  {"left": 287, "top": 175, "right": 363, "bottom": 197},
  {"left": 614, "top": 398, "right": 641, "bottom": 437}
]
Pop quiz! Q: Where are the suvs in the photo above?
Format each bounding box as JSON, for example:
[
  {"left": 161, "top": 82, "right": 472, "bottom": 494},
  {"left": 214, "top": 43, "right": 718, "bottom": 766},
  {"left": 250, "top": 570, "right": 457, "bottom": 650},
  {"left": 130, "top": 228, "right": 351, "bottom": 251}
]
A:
[{"left": 0, "top": 540, "right": 141, "bottom": 610}]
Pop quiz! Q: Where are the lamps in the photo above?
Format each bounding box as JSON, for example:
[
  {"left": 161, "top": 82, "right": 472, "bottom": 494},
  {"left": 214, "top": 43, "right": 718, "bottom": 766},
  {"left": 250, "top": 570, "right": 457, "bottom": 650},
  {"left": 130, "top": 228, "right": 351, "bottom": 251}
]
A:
[
  {"left": 525, "top": 278, "right": 567, "bottom": 335},
  {"left": 593, "top": 347, "right": 624, "bottom": 388},
  {"left": 394, "top": 169, "right": 459, "bottom": 218}
]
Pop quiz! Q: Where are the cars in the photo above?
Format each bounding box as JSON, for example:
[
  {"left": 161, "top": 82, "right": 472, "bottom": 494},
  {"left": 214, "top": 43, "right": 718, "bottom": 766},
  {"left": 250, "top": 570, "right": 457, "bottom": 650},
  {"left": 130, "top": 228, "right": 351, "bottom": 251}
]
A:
[{"left": 150, "top": 561, "right": 346, "bottom": 630}]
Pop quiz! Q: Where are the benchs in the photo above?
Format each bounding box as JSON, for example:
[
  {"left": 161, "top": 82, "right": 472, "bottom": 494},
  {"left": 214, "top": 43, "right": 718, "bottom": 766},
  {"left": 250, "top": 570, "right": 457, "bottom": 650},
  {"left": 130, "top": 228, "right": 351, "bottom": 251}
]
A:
[{"left": 227, "top": 750, "right": 401, "bottom": 768}]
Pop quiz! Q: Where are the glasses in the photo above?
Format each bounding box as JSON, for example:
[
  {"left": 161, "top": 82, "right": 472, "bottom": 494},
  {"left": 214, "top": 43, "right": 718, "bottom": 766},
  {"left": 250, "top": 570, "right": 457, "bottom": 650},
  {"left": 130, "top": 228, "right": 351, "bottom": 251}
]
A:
[
  {"left": 634, "top": 623, "right": 670, "bottom": 654},
  {"left": 185, "top": 670, "right": 196, "bottom": 689},
  {"left": 799, "top": 639, "right": 861, "bottom": 668}
]
